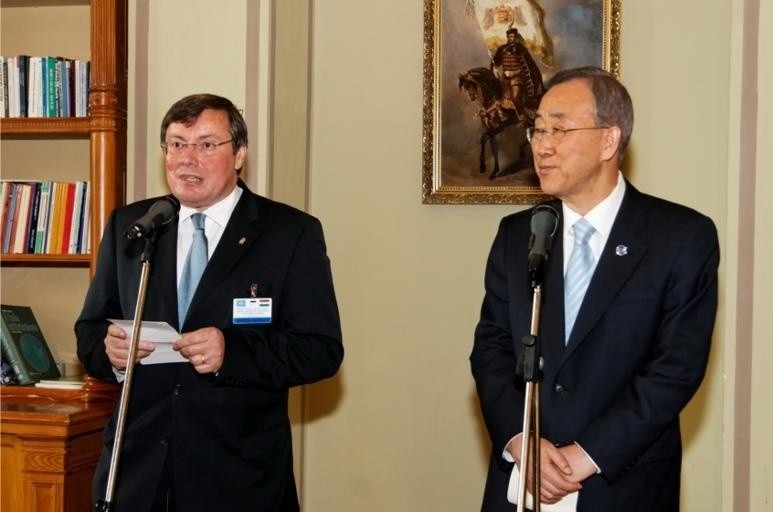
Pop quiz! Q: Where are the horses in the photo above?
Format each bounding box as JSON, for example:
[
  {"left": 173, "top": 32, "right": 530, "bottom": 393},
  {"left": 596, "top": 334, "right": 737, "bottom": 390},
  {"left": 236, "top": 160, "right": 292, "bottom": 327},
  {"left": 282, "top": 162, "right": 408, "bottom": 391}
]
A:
[{"left": 458, "top": 65, "right": 549, "bottom": 181}]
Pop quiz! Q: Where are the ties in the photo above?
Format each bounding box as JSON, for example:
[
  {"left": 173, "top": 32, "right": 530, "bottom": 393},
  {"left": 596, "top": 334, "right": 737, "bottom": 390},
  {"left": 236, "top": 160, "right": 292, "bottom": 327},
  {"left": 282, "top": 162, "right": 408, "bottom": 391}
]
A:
[
  {"left": 563, "top": 220, "right": 598, "bottom": 347},
  {"left": 177, "top": 213, "right": 209, "bottom": 332}
]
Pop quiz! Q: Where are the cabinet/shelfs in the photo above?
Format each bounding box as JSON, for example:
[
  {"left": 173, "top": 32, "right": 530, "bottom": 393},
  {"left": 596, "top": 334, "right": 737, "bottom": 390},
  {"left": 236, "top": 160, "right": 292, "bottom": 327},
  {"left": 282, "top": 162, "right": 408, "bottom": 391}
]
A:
[{"left": 0, "top": 0, "right": 128, "bottom": 512}]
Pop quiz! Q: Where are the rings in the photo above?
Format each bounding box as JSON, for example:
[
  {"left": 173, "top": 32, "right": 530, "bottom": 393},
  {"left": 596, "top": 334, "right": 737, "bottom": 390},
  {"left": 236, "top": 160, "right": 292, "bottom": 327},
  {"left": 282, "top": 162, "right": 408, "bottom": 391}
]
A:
[{"left": 200, "top": 353, "right": 206, "bottom": 365}]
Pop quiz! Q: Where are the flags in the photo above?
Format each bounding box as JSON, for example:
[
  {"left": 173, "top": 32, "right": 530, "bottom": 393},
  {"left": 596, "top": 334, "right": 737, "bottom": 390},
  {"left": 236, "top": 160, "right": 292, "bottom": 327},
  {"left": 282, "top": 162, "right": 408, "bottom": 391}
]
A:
[{"left": 472, "top": 1, "right": 560, "bottom": 72}]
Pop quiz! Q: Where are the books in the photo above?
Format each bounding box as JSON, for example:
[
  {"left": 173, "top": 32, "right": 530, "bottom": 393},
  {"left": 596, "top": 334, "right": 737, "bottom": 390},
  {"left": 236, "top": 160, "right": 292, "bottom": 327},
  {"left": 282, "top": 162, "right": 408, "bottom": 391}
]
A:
[
  {"left": 39, "top": 376, "right": 85, "bottom": 385},
  {"left": 0, "top": 303, "right": 61, "bottom": 385},
  {"left": 0, "top": 51, "right": 89, "bottom": 258},
  {"left": 34, "top": 382, "right": 82, "bottom": 391},
  {"left": 0, "top": 342, "right": 18, "bottom": 386}
]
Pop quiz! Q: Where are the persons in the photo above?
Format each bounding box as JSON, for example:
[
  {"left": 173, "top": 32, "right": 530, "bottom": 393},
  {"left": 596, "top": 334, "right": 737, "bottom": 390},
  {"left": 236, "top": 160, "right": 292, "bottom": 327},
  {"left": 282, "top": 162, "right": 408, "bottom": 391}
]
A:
[
  {"left": 464, "top": 62, "right": 724, "bottom": 512},
  {"left": 70, "top": 91, "right": 346, "bottom": 512},
  {"left": 486, "top": 27, "right": 543, "bottom": 126}
]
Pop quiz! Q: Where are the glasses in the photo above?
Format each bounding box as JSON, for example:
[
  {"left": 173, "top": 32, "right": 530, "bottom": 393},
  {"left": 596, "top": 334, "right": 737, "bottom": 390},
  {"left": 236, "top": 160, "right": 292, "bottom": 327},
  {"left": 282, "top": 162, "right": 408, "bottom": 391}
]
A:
[
  {"left": 525, "top": 124, "right": 609, "bottom": 144},
  {"left": 159, "top": 138, "right": 236, "bottom": 155}
]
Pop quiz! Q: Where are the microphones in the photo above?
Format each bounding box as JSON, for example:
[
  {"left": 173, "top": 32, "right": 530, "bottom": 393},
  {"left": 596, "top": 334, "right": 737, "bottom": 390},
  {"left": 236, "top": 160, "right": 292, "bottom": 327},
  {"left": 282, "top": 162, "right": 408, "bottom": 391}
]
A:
[
  {"left": 528, "top": 204, "right": 561, "bottom": 288},
  {"left": 121, "top": 195, "right": 181, "bottom": 241}
]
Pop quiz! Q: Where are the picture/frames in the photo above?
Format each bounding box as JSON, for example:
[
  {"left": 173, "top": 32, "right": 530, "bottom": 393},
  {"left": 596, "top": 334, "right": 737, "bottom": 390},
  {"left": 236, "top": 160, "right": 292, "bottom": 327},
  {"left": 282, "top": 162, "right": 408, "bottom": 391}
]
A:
[{"left": 423, "top": 1, "right": 620, "bottom": 205}]
